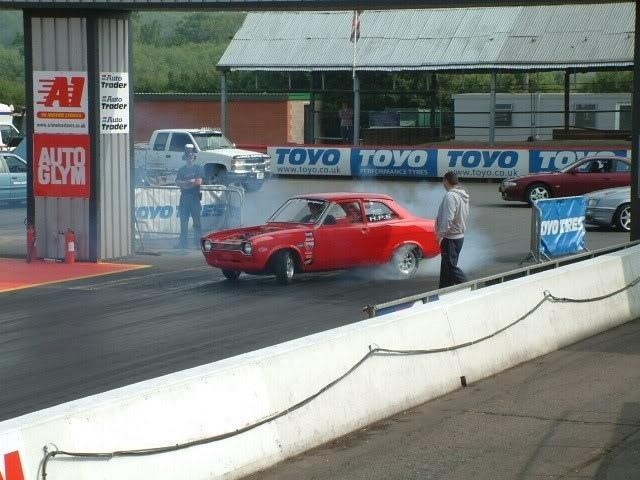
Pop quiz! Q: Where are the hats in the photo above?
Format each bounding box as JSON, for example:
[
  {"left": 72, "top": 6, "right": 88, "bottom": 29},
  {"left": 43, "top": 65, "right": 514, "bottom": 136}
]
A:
[{"left": 445, "top": 172, "right": 458, "bottom": 185}]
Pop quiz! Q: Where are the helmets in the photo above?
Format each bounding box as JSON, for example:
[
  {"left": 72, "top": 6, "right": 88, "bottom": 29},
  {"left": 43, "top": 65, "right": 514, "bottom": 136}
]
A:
[{"left": 182, "top": 151, "right": 196, "bottom": 161}]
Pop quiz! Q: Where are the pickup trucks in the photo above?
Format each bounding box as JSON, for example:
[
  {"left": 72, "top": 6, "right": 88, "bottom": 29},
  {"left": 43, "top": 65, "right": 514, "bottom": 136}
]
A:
[{"left": 133, "top": 127, "right": 272, "bottom": 192}]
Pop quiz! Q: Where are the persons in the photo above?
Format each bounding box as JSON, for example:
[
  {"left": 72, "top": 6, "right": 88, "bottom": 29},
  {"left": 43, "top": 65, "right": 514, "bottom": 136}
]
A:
[
  {"left": 587, "top": 161, "right": 602, "bottom": 173},
  {"left": 435, "top": 171, "right": 469, "bottom": 289},
  {"left": 299, "top": 200, "right": 336, "bottom": 225},
  {"left": 175, "top": 150, "right": 203, "bottom": 249},
  {"left": 339, "top": 99, "right": 354, "bottom": 144}
]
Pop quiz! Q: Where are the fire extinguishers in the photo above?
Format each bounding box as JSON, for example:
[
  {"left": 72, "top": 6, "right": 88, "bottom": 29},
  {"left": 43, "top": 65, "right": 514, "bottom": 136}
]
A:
[
  {"left": 24, "top": 217, "right": 36, "bottom": 262},
  {"left": 64, "top": 224, "right": 77, "bottom": 264}
]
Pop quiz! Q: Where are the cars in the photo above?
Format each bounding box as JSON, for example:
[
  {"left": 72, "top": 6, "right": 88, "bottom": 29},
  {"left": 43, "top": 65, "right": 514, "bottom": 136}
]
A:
[
  {"left": 497, "top": 153, "right": 634, "bottom": 208},
  {"left": 199, "top": 191, "right": 444, "bottom": 287},
  {"left": 0, "top": 102, "right": 29, "bottom": 208},
  {"left": 577, "top": 182, "right": 632, "bottom": 234}
]
